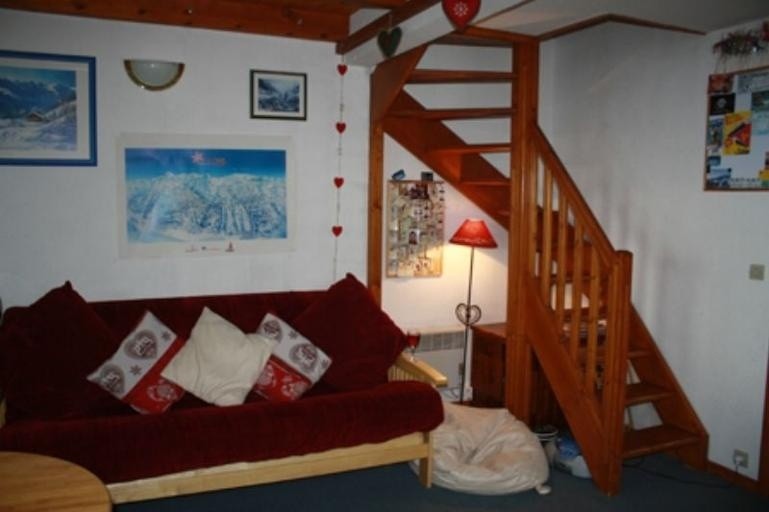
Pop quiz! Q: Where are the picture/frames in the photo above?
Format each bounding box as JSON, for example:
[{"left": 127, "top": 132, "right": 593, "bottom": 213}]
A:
[
  {"left": 0, "top": 49, "right": 98, "bottom": 166},
  {"left": 249, "top": 70, "right": 307, "bottom": 120}
]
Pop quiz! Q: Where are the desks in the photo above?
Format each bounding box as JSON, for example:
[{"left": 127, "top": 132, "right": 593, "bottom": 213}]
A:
[
  {"left": 1, "top": 451, "right": 113, "bottom": 512},
  {"left": 469, "top": 318, "right": 607, "bottom": 437}
]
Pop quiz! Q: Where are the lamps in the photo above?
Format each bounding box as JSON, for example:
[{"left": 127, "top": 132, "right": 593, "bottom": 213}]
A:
[
  {"left": 448, "top": 218, "right": 498, "bottom": 404},
  {"left": 124, "top": 59, "right": 185, "bottom": 91}
]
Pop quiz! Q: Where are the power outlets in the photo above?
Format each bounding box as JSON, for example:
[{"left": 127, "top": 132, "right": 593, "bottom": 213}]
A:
[{"left": 733, "top": 449, "right": 749, "bottom": 468}]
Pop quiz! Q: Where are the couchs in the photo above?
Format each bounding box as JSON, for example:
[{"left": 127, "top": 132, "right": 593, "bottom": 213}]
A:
[{"left": 1, "top": 288, "right": 448, "bottom": 505}]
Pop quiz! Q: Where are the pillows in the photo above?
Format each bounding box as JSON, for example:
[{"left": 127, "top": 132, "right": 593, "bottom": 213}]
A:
[
  {"left": 85, "top": 310, "right": 189, "bottom": 414},
  {"left": 159, "top": 306, "right": 277, "bottom": 408},
  {"left": 293, "top": 272, "right": 407, "bottom": 392},
  {"left": 253, "top": 312, "right": 335, "bottom": 401},
  {"left": 1, "top": 281, "right": 123, "bottom": 421}
]
